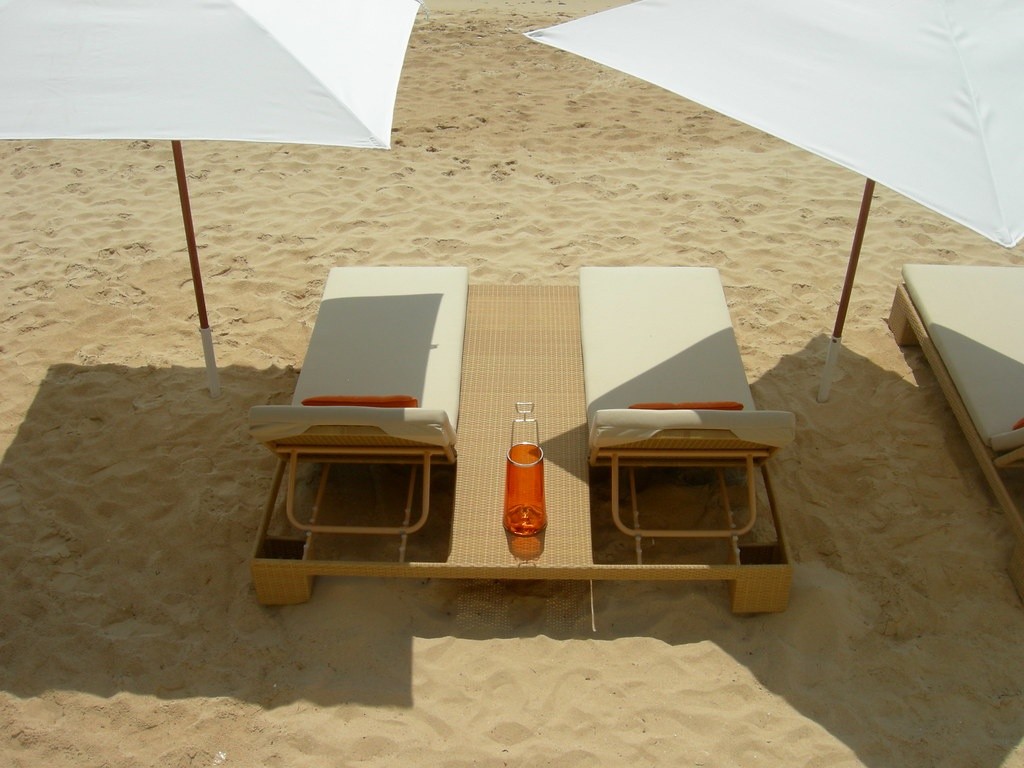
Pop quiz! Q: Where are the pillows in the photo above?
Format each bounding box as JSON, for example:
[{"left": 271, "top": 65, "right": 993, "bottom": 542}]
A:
[
  {"left": 300, "top": 395, "right": 418, "bottom": 407},
  {"left": 628, "top": 401, "right": 745, "bottom": 411}
]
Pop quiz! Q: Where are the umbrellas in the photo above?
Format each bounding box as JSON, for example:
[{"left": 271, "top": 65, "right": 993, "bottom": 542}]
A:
[
  {"left": 0, "top": 0, "right": 430, "bottom": 402},
  {"left": 520, "top": 0, "right": 1024, "bottom": 404}
]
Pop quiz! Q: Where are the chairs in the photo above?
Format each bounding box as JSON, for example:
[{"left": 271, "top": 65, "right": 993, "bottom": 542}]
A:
[
  {"left": 889, "top": 263, "right": 1024, "bottom": 472},
  {"left": 580, "top": 265, "right": 796, "bottom": 538},
  {"left": 248, "top": 265, "right": 469, "bottom": 534}
]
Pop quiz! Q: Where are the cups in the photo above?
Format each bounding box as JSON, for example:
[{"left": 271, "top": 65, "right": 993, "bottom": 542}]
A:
[{"left": 502, "top": 442, "right": 548, "bottom": 538}]
